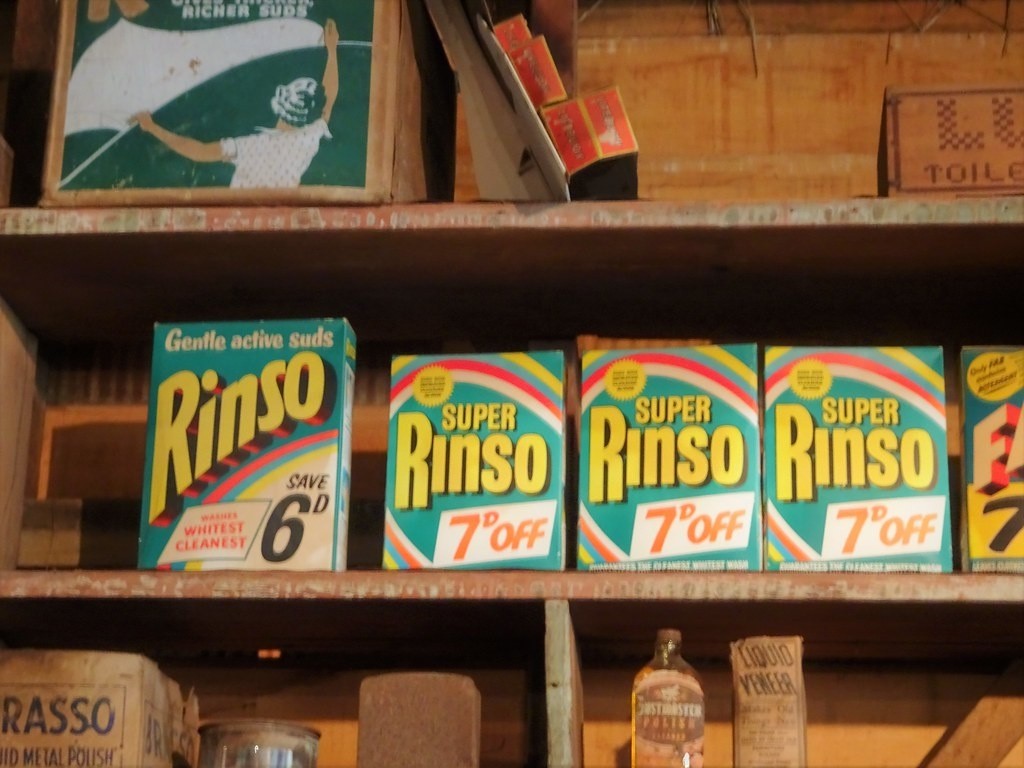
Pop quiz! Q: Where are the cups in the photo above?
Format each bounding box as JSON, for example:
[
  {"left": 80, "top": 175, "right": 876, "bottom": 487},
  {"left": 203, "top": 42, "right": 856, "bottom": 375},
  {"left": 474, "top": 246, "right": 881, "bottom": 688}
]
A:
[{"left": 195, "top": 720, "right": 321, "bottom": 768}]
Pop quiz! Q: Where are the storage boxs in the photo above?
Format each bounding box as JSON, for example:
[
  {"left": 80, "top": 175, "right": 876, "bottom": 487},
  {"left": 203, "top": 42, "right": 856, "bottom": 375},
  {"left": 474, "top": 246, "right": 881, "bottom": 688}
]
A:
[
  {"left": 38, "top": 0, "right": 438, "bottom": 206},
  {"left": 141, "top": 310, "right": 1024, "bottom": 577}
]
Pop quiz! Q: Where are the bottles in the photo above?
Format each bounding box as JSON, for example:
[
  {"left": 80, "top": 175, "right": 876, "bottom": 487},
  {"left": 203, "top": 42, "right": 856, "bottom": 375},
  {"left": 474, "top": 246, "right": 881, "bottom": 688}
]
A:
[{"left": 630, "top": 627, "right": 706, "bottom": 768}]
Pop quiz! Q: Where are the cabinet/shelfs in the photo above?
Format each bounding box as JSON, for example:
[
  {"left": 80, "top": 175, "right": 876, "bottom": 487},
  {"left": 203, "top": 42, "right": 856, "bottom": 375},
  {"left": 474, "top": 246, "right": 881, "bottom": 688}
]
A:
[{"left": 0, "top": 0, "right": 1024, "bottom": 767}]
